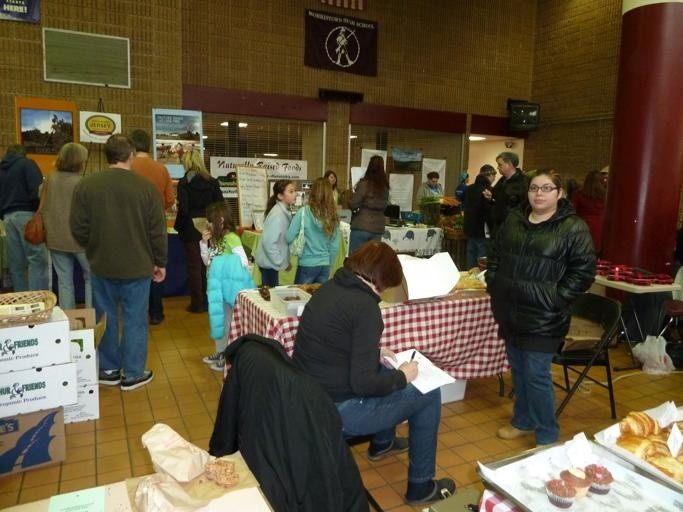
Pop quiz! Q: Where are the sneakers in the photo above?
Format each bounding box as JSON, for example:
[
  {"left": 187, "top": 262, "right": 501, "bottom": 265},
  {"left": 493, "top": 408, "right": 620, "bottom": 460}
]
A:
[
  {"left": 149, "top": 314, "right": 165, "bottom": 325},
  {"left": 209, "top": 358, "right": 226, "bottom": 371},
  {"left": 496, "top": 423, "right": 535, "bottom": 440},
  {"left": 120, "top": 369, "right": 154, "bottom": 391},
  {"left": 186, "top": 303, "right": 208, "bottom": 314},
  {"left": 99, "top": 368, "right": 122, "bottom": 386},
  {"left": 202, "top": 351, "right": 220, "bottom": 364}
]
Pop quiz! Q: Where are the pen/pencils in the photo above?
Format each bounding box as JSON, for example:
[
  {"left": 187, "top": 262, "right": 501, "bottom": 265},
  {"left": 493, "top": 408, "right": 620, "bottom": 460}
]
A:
[{"left": 410, "top": 351, "right": 416, "bottom": 362}]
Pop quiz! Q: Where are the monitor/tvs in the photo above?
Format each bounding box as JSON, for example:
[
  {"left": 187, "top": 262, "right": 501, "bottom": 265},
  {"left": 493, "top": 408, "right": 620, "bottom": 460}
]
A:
[{"left": 506, "top": 99, "right": 540, "bottom": 130}]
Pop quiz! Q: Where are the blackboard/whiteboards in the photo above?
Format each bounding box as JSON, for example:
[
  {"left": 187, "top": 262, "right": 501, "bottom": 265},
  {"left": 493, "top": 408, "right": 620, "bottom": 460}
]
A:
[{"left": 235, "top": 165, "right": 270, "bottom": 229}]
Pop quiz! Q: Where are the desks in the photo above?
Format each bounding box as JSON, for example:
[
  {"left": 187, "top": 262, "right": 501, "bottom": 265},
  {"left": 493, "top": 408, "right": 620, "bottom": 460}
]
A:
[
  {"left": 339, "top": 216, "right": 442, "bottom": 257},
  {"left": 235, "top": 228, "right": 346, "bottom": 286},
  {"left": 159, "top": 226, "right": 190, "bottom": 297}
]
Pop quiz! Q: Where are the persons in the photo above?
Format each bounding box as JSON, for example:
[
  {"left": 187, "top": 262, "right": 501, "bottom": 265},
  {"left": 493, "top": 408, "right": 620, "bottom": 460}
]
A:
[
  {"left": 291, "top": 239, "right": 456, "bottom": 507},
  {"left": 173, "top": 150, "right": 390, "bottom": 372},
  {"left": 1, "top": 129, "right": 175, "bottom": 391},
  {"left": 416, "top": 152, "right": 609, "bottom": 447}
]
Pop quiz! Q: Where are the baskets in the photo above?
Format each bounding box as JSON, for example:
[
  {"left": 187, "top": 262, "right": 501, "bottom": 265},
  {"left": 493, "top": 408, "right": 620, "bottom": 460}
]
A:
[{"left": 0, "top": 288, "right": 58, "bottom": 330}]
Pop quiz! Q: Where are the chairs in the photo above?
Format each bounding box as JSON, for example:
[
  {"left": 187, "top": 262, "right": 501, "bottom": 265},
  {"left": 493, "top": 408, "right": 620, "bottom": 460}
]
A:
[
  {"left": 240, "top": 341, "right": 397, "bottom": 512},
  {"left": 508, "top": 291, "right": 622, "bottom": 422}
]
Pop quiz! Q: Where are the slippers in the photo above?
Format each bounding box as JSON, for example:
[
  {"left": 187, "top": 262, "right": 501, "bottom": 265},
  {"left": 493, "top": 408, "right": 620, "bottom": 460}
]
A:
[
  {"left": 367, "top": 437, "right": 409, "bottom": 461},
  {"left": 404, "top": 478, "right": 456, "bottom": 506}
]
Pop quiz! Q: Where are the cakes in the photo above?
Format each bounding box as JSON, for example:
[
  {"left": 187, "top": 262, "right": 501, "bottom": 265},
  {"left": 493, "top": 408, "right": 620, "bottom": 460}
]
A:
[{"left": 546, "top": 464, "right": 614, "bottom": 508}]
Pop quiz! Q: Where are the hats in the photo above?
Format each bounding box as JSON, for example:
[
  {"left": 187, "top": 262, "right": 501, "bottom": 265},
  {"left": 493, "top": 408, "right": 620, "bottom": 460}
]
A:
[
  {"left": 460, "top": 172, "right": 467, "bottom": 182},
  {"left": 479, "top": 164, "right": 497, "bottom": 177}
]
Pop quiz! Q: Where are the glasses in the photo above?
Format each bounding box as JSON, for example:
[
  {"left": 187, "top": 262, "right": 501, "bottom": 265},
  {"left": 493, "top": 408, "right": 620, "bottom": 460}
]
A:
[{"left": 527, "top": 185, "right": 559, "bottom": 192}]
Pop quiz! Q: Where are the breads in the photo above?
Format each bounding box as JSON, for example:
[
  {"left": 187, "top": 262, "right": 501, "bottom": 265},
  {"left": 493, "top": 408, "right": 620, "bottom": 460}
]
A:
[{"left": 616, "top": 410, "right": 683, "bottom": 483}]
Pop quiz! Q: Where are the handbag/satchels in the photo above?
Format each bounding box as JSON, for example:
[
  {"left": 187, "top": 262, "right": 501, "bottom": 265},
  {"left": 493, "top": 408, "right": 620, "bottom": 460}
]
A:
[
  {"left": 288, "top": 206, "right": 306, "bottom": 258},
  {"left": 23, "top": 208, "right": 46, "bottom": 245}
]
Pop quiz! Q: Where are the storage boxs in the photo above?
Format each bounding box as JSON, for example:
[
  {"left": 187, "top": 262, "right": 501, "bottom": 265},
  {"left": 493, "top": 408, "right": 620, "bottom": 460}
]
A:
[{"left": 0, "top": 308, "right": 108, "bottom": 479}]
